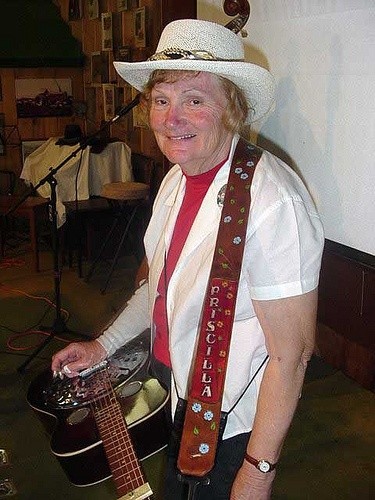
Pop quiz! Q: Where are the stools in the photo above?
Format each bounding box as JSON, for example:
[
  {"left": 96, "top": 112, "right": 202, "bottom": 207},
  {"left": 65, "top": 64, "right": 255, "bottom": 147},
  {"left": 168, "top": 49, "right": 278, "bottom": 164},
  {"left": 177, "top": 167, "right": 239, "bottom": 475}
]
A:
[
  {"left": 1, "top": 196, "right": 51, "bottom": 273},
  {"left": 83, "top": 180, "right": 151, "bottom": 297}
]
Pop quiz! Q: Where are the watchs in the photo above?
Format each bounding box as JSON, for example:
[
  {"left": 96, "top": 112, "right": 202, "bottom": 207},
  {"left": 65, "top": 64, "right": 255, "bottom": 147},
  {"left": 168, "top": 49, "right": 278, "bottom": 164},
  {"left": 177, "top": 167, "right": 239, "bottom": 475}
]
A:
[{"left": 245, "top": 454, "right": 278, "bottom": 473}]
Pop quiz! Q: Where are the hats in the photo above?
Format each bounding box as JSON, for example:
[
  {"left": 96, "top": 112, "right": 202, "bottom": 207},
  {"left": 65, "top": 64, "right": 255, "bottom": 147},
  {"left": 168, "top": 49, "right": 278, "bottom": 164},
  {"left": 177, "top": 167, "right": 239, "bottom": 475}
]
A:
[{"left": 113, "top": 19, "right": 277, "bottom": 125}]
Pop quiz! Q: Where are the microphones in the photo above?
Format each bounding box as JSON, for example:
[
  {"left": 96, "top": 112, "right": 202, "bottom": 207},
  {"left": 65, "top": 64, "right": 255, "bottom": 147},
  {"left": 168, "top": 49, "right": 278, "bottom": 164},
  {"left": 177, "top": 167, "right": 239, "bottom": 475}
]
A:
[{"left": 106, "top": 92, "right": 143, "bottom": 127}]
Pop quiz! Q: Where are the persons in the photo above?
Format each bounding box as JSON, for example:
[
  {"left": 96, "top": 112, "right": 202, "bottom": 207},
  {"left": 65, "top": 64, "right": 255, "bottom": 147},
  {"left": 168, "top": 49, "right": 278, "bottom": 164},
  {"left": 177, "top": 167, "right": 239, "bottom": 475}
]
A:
[{"left": 48, "top": 18, "right": 325, "bottom": 500}]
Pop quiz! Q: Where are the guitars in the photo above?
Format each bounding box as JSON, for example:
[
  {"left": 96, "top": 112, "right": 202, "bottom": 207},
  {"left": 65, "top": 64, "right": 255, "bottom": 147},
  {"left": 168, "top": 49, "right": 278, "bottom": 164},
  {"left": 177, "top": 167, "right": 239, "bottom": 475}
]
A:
[{"left": 25, "top": 339, "right": 170, "bottom": 500}]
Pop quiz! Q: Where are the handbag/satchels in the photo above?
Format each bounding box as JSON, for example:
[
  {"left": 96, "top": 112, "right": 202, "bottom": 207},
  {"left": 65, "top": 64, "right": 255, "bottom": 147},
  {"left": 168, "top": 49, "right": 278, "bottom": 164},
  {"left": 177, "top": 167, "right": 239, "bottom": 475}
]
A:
[{"left": 167, "top": 399, "right": 228, "bottom": 485}]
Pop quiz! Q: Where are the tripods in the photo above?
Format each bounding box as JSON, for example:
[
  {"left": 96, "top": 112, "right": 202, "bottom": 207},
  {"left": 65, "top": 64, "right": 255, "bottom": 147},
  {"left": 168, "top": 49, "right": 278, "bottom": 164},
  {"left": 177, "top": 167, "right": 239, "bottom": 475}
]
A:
[{"left": 3, "top": 127, "right": 106, "bottom": 372}]
